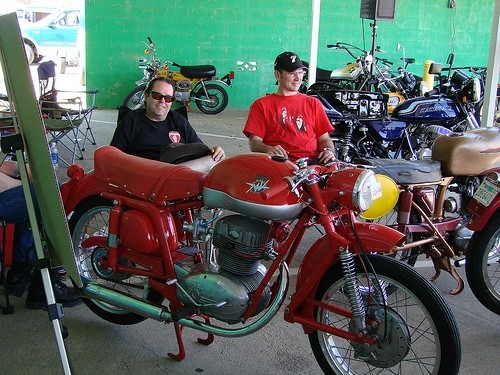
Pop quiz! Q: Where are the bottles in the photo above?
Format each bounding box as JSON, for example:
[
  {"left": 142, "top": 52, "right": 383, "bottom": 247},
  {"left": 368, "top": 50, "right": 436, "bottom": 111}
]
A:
[{"left": 50, "top": 142, "right": 59, "bottom": 170}]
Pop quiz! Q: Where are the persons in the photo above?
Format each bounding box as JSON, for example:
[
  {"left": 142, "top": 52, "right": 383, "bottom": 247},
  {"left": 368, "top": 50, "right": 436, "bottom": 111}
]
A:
[
  {"left": 110, "top": 77, "right": 225, "bottom": 172},
  {"left": 242, "top": 52, "right": 339, "bottom": 165},
  {"left": 0, "top": 161, "right": 84, "bottom": 311}
]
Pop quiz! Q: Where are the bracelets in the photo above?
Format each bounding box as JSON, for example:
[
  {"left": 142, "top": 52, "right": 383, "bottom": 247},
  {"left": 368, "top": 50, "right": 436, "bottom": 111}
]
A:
[{"left": 324, "top": 148, "right": 336, "bottom": 155}]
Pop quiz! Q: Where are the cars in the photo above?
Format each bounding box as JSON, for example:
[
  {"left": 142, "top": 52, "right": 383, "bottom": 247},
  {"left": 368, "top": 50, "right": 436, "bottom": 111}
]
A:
[{"left": 17, "top": 4, "right": 85, "bottom": 66}]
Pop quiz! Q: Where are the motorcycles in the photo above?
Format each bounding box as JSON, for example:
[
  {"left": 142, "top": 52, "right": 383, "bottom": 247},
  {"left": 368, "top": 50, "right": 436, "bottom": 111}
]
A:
[
  {"left": 122, "top": 36, "right": 235, "bottom": 116},
  {"left": 59, "top": 144, "right": 463, "bottom": 375},
  {"left": 274, "top": 40, "right": 500, "bottom": 319}
]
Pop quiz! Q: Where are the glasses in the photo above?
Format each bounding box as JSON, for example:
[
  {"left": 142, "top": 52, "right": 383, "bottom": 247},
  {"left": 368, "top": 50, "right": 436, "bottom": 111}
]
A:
[
  {"left": 281, "top": 71, "right": 305, "bottom": 76},
  {"left": 149, "top": 91, "right": 175, "bottom": 103}
]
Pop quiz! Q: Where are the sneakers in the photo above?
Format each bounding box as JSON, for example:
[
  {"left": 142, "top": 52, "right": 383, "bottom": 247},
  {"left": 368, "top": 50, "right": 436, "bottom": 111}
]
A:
[
  {"left": 6, "top": 269, "right": 24, "bottom": 292},
  {"left": 26, "top": 275, "right": 82, "bottom": 310}
]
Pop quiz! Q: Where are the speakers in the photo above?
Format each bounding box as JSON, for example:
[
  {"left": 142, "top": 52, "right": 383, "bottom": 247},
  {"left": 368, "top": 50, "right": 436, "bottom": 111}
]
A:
[{"left": 360, "top": 0, "right": 397, "bottom": 21}]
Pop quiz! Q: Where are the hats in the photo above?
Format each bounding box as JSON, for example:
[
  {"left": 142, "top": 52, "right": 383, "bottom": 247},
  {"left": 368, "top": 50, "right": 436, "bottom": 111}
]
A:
[{"left": 274, "top": 52, "right": 307, "bottom": 73}]
